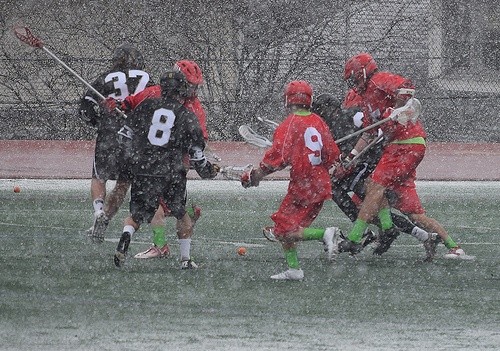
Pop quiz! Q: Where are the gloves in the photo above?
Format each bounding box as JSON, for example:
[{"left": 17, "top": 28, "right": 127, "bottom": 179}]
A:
[
  {"left": 380, "top": 111, "right": 406, "bottom": 139},
  {"left": 334, "top": 154, "right": 357, "bottom": 179},
  {"left": 241, "top": 167, "right": 257, "bottom": 188},
  {"left": 194, "top": 159, "right": 218, "bottom": 178},
  {"left": 100, "top": 97, "right": 122, "bottom": 118}
]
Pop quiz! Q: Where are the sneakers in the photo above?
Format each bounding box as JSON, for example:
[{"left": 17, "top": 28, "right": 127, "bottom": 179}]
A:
[
  {"left": 373, "top": 224, "right": 400, "bottom": 255},
  {"left": 443, "top": 245, "right": 478, "bottom": 260},
  {"left": 92, "top": 211, "right": 109, "bottom": 243},
  {"left": 325, "top": 230, "right": 363, "bottom": 255},
  {"left": 191, "top": 207, "right": 201, "bottom": 227},
  {"left": 322, "top": 226, "right": 341, "bottom": 261},
  {"left": 270, "top": 268, "right": 304, "bottom": 280},
  {"left": 133, "top": 243, "right": 170, "bottom": 259},
  {"left": 421, "top": 232, "right": 441, "bottom": 262},
  {"left": 262, "top": 227, "right": 281, "bottom": 242},
  {"left": 181, "top": 260, "right": 198, "bottom": 269},
  {"left": 114, "top": 232, "right": 132, "bottom": 267},
  {"left": 360, "top": 231, "right": 377, "bottom": 247}
]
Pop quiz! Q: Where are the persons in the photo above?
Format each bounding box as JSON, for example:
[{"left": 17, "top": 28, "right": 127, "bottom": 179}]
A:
[
  {"left": 324, "top": 53, "right": 466, "bottom": 256},
  {"left": 311, "top": 93, "right": 442, "bottom": 261},
  {"left": 113, "top": 72, "right": 221, "bottom": 271},
  {"left": 78, "top": 45, "right": 156, "bottom": 243},
  {"left": 101, "top": 58, "right": 209, "bottom": 258},
  {"left": 344, "top": 88, "right": 384, "bottom": 212},
  {"left": 240, "top": 79, "right": 341, "bottom": 281}
]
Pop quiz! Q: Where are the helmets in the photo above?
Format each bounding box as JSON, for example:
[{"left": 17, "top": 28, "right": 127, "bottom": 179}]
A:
[
  {"left": 312, "top": 93, "right": 341, "bottom": 122},
  {"left": 159, "top": 71, "right": 188, "bottom": 99},
  {"left": 111, "top": 44, "right": 146, "bottom": 71},
  {"left": 173, "top": 59, "right": 202, "bottom": 84},
  {"left": 283, "top": 80, "right": 313, "bottom": 106},
  {"left": 341, "top": 54, "right": 378, "bottom": 95}
]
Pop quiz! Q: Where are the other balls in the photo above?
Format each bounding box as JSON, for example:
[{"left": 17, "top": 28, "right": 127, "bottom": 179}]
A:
[
  {"left": 237, "top": 246, "right": 246, "bottom": 256},
  {"left": 14, "top": 187, "right": 20, "bottom": 193}
]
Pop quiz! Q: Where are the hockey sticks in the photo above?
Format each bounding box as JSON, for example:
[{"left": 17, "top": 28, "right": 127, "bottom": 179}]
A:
[
  {"left": 183, "top": 164, "right": 255, "bottom": 184},
  {"left": 14, "top": 26, "right": 127, "bottom": 119},
  {"left": 241, "top": 97, "right": 422, "bottom": 189},
  {"left": 254, "top": 113, "right": 280, "bottom": 129},
  {"left": 262, "top": 133, "right": 385, "bottom": 243},
  {"left": 237, "top": 125, "right": 273, "bottom": 147}
]
